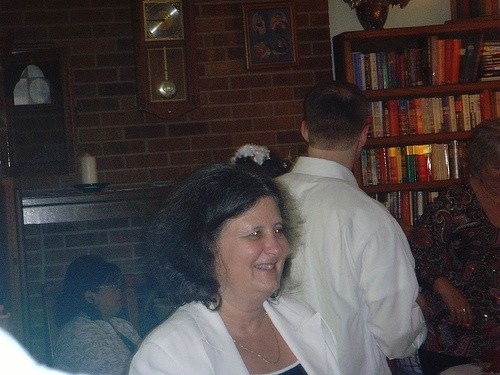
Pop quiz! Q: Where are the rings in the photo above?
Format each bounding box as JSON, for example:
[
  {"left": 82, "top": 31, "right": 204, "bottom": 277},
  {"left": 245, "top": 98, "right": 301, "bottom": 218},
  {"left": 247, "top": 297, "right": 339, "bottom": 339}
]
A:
[{"left": 462, "top": 308, "right": 467, "bottom": 313}]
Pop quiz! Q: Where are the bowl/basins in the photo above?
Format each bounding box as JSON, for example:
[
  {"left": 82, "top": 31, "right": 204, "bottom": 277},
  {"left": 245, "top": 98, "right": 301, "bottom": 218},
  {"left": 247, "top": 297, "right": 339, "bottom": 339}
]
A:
[{"left": 76, "top": 183, "right": 109, "bottom": 192}]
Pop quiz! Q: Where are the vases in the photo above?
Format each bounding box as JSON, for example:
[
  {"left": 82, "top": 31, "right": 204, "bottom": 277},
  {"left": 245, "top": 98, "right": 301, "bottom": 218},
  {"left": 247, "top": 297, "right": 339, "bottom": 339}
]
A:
[{"left": 356, "top": 0, "right": 389, "bottom": 30}]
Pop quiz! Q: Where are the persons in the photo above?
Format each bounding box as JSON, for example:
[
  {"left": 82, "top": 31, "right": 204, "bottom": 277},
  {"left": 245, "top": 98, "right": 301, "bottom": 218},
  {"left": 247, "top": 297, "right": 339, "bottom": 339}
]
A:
[
  {"left": 230, "top": 142, "right": 287, "bottom": 185},
  {"left": 51, "top": 255, "right": 143, "bottom": 375},
  {"left": 263, "top": 79, "right": 428, "bottom": 375},
  {"left": 406, "top": 118, "right": 499, "bottom": 374},
  {"left": 127, "top": 164, "right": 343, "bottom": 375}
]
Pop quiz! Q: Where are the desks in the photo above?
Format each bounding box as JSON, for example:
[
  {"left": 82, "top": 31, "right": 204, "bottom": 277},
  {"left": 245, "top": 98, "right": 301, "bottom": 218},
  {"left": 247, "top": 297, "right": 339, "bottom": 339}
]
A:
[{"left": 18, "top": 181, "right": 186, "bottom": 363}]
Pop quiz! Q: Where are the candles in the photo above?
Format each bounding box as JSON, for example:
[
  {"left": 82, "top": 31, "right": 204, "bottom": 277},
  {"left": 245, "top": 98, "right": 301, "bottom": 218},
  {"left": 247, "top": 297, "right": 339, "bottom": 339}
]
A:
[{"left": 80, "top": 152, "right": 99, "bottom": 184}]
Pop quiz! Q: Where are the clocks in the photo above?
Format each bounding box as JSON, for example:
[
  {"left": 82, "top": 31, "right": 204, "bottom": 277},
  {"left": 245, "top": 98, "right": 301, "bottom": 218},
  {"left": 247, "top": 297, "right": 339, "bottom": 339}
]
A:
[{"left": 132, "top": 0, "right": 200, "bottom": 119}]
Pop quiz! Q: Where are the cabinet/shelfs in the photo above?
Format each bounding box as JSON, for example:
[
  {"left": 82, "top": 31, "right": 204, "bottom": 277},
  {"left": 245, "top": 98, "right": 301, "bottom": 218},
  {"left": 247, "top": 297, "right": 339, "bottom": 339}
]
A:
[{"left": 332, "top": 15, "right": 500, "bottom": 239}]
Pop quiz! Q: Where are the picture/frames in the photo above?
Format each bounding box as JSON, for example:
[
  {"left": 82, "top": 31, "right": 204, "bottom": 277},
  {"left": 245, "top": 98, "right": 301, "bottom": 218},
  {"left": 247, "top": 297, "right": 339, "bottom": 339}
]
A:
[{"left": 241, "top": 3, "right": 301, "bottom": 71}]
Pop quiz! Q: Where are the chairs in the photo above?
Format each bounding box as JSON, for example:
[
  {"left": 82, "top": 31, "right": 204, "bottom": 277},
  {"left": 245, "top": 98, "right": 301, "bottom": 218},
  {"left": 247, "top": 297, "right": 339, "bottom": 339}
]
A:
[{"left": 40, "top": 281, "right": 138, "bottom": 367}]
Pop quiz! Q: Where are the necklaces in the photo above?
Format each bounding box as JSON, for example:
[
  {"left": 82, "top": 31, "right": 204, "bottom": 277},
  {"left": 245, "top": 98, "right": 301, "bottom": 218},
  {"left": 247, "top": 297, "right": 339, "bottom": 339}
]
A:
[{"left": 233, "top": 313, "right": 281, "bottom": 364}]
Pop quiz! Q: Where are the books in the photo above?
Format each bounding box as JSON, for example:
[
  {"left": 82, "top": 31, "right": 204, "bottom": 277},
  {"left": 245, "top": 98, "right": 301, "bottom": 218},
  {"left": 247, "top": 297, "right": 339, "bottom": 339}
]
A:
[
  {"left": 351, "top": 37, "right": 476, "bottom": 91},
  {"left": 479, "top": 42, "right": 500, "bottom": 81},
  {"left": 366, "top": 94, "right": 484, "bottom": 140},
  {"left": 354, "top": 141, "right": 468, "bottom": 186},
  {"left": 366, "top": 190, "right": 448, "bottom": 228},
  {"left": 491, "top": 91, "right": 500, "bottom": 120}
]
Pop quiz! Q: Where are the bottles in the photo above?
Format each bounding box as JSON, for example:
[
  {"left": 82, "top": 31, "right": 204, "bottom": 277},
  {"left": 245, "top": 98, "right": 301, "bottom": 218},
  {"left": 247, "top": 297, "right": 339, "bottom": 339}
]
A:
[{"left": 81, "top": 153, "right": 97, "bottom": 183}]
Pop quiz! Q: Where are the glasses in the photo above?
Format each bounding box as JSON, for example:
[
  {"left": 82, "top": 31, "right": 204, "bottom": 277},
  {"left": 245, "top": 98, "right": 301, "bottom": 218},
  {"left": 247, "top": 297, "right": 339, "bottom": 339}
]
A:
[{"left": 92, "top": 283, "right": 119, "bottom": 291}]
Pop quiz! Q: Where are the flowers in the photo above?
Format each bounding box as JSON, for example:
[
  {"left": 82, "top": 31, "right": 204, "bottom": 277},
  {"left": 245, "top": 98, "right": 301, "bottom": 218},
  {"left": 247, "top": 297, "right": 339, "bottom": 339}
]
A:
[{"left": 343, "top": 0, "right": 409, "bottom": 11}]
